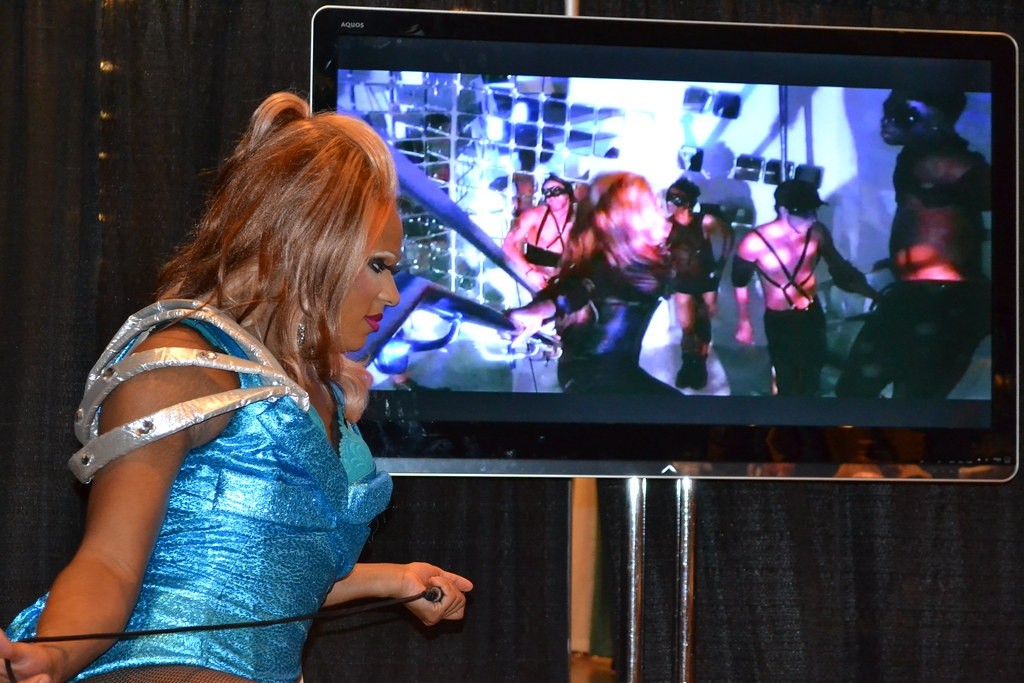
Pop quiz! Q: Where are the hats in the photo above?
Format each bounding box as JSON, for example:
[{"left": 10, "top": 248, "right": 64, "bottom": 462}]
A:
[
  {"left": 774, "top": 180, "right": 831, "bottom": 208},
  {"left": 673, "top": 176, "right": 701, "bottom": 208}
]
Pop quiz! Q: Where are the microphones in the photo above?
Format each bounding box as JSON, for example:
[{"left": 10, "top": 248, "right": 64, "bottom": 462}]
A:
[{"left": 423, "top": 586, "right": 444, "bottom": 602}]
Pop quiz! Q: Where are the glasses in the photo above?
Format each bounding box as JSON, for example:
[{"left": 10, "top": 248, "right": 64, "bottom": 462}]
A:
[
  {"left": 666, "top": 189, "right": 687, "bottom": 207},
  {"left": 885, "top": 111, "right": 917, "bottom": 130},
  {"left": 787, "top": 206, "right": 818, "bottom": 218},
  {"left": 545, "top": 187, "right": 567, "bottom": 198}
]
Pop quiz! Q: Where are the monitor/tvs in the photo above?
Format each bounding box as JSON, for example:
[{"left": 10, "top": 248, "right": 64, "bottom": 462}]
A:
[{"left": 307, "top": 5, "right": 1018, "bottom": 480}]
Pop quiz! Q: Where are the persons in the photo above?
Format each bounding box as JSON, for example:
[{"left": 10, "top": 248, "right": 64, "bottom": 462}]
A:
[
  {"left": 0, "top": 93, "right": 473, "bottom": 683},
  {"left": 500, "top": 76, "right": 992, "bottom": 421}
]
